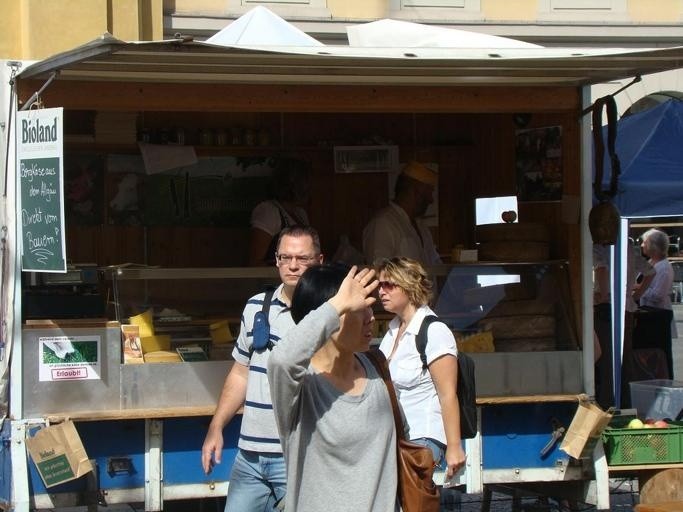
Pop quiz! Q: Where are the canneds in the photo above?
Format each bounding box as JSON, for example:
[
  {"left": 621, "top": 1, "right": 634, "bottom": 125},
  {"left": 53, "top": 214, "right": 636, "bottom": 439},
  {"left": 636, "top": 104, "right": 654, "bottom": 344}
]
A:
[{"left": 141, "top": 127, "right": 270, "bottom": 145}]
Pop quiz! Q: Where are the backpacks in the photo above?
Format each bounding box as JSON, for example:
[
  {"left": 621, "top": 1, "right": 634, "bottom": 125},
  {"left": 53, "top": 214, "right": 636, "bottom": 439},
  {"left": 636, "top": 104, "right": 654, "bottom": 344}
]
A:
[{"left": 416, "top": 315, "right": 478, "bottom": 439}]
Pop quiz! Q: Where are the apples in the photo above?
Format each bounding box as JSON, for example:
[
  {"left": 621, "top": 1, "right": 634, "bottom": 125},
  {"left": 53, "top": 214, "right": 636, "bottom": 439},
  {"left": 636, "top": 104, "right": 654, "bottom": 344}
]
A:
[{"left": 622, "top": 419, "right": 669, "bottom": 429}]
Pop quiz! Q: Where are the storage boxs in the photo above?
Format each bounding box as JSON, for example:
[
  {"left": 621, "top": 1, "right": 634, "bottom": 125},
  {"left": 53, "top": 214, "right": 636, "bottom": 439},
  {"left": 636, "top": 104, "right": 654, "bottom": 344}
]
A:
[
  {"left": 629, "top": 379, "right": 683, "bottom": 422},
  {"left": 602, "top": 416, "right": 682, "bottom": 466}
]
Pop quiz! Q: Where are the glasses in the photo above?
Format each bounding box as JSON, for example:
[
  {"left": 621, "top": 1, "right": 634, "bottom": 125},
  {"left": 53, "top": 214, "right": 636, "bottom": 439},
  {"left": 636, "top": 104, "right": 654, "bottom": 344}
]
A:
[
  {"left": 378, "top": 281, "right": 399, "bottom": 290},
  {"left": 276, "top": 253, "right": 317, "bottom": 265}
]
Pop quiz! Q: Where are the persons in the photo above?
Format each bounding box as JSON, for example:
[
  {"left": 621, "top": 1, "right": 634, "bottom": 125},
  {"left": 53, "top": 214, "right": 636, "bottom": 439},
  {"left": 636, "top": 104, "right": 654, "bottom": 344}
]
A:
[
  {"left": 369, "top": 257, "right": 469, "bottom": 512},
  {"left": 593, "top": 325, "right": 603, "bottom": 366},
  {"left": 248, "top": 158, "right": 315, "bottom": 266},
  {"left": 592, "top": 234, "right": 656, "bottom": 407},
  {"left": 265, "top": 263, "right": 415, "bottom": 512},
  {"left": 635, "top": 226, "right": 673, "bottom": 385},
  {"left": 359, "top": 160, "right": 444, "bottom": 266},
  {"left": 199, "top": 224, "right": 320, "bottom": 512}
]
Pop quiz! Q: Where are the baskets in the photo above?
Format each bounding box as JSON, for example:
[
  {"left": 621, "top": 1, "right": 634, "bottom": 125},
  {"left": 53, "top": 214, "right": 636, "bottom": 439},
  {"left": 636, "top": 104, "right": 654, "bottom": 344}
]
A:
[{"left": 602, "top": 424, "right": 683, "bottom": 466}]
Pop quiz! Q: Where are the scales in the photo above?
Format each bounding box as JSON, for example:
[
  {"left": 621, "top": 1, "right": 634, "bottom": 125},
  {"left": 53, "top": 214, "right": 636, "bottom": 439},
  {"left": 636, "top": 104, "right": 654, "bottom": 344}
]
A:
[{"left": 42, "top": 262, "right": 98, "bottom": 286}]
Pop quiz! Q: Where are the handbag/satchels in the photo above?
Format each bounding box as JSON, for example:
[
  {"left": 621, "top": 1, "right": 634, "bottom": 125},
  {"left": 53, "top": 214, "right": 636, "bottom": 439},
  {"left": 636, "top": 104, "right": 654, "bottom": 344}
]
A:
[
  {"left": 397, "top": 440, "right": 441, "bottom": 512},
  {"left": 558, "top": 394, "right": 613, "bottom": 459},
  {"left": 24, "top": 415, "right": 94, "bottom": 490}
]
